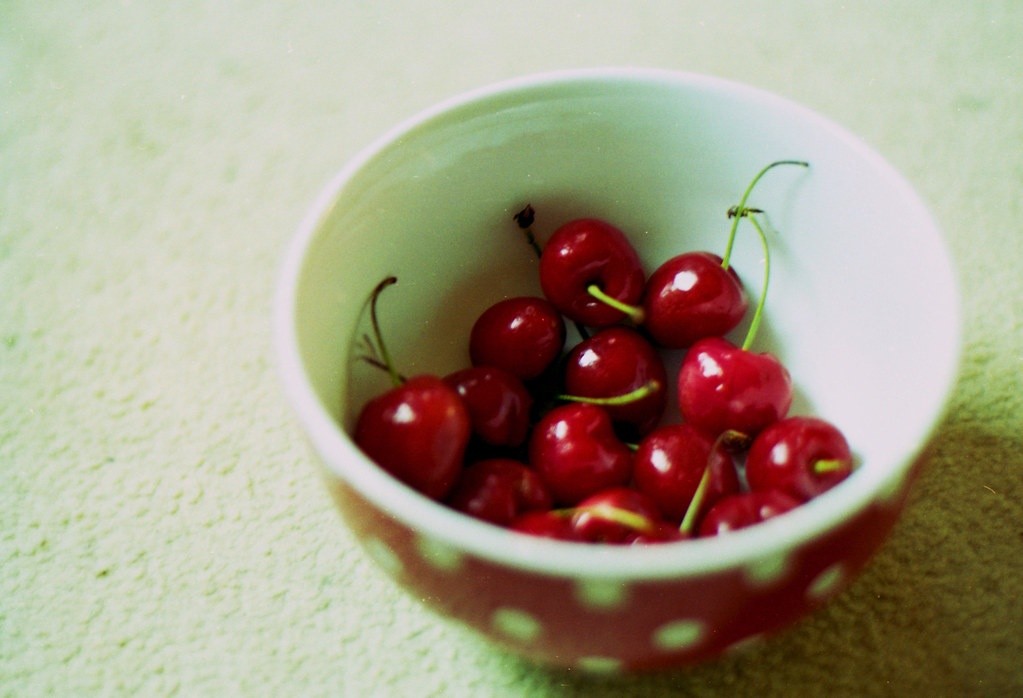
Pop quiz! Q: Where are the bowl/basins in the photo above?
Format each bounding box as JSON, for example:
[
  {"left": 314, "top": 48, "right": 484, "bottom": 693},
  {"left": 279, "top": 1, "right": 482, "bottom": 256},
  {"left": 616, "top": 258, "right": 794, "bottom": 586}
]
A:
[{"left": 274, "top": 66, "right": 964, "bottom": 676}]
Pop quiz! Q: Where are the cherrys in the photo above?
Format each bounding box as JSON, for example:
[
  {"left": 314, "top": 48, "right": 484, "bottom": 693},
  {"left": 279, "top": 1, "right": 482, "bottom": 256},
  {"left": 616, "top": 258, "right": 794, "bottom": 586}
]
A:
[{"left": 348, "top": 158, "right": 857, "bottom": 545}]
